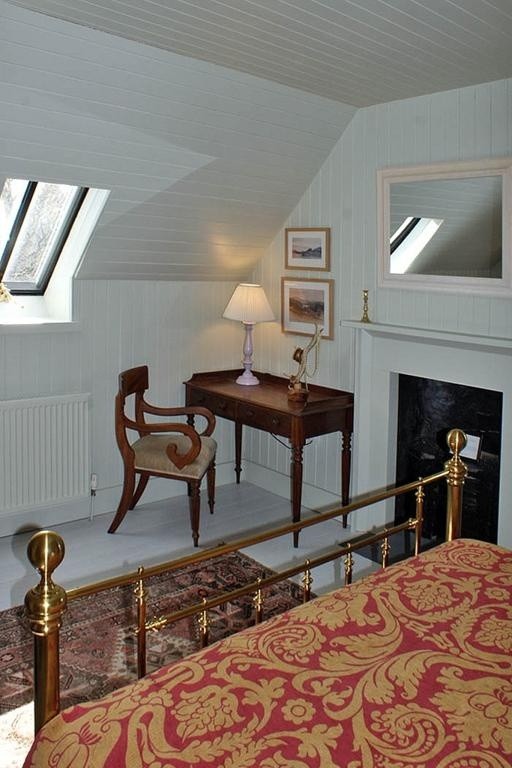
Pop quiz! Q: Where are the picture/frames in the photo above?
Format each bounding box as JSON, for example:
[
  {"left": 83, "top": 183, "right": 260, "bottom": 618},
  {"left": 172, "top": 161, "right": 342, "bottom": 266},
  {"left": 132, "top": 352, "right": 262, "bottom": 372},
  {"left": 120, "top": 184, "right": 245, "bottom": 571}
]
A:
[{"left": 280, "top": 227, "right": 335, "bottom": 341}]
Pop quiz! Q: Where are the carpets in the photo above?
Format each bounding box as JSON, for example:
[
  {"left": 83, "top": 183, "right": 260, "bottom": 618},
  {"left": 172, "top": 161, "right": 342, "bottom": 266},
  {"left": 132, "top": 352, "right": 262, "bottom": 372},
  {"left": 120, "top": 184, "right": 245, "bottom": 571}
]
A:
[{"left": 0, "top": 542, "right": 317, "bottom": 767}]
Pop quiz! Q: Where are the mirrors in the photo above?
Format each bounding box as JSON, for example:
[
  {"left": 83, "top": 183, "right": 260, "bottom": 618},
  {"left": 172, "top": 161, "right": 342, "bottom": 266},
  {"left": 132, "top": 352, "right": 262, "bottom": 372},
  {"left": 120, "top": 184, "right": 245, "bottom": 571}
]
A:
[{"left": 376, "top": 156, "right": 512, "bottom": 300}]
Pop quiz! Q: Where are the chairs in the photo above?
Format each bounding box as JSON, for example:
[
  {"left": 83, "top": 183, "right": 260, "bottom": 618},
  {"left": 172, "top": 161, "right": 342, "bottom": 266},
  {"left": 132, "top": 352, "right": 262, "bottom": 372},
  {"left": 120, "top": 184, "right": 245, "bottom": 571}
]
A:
[{"left": 105, "top": 365, "right": 217, "bottom": 547}]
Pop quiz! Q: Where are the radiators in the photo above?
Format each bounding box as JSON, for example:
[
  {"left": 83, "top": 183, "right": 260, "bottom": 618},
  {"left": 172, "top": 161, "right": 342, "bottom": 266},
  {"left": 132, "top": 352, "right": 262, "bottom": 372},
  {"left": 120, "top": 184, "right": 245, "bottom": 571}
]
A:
[{"left": 0, "top": 392, "right": 99, "bottom": 521}]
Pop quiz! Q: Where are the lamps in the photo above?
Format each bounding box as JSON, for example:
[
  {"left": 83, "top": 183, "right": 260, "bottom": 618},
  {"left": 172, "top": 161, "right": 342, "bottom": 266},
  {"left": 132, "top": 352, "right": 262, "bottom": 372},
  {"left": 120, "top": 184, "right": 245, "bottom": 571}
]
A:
[{"left": 220, "top": 282, "right": 275, "bottom": 387}]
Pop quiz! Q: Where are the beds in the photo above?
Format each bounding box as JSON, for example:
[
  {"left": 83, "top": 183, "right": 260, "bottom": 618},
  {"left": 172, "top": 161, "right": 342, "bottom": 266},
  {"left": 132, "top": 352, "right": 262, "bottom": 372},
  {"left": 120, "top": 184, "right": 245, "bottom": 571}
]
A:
[{"left": 23, "top": 427, "right": 512, "bottom": 768}]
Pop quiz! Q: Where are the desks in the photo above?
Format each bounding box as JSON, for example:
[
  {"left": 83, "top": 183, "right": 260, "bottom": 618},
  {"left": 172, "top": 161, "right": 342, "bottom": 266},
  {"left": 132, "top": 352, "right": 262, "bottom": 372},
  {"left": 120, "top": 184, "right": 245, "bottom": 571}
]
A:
[{"left": 183, "top": 368, "right": 354, "bottom": 549}]
